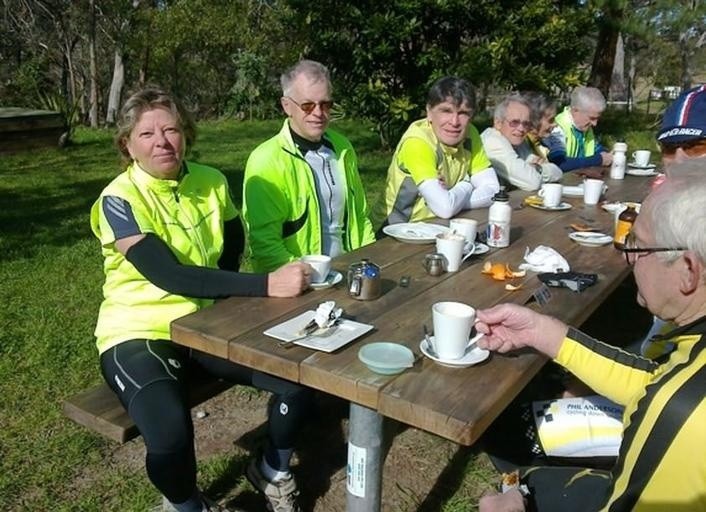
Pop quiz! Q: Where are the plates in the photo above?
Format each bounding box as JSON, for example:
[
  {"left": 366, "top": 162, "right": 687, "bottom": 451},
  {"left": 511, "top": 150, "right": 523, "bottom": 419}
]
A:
[
  {"left": 305, "top": 271, "right": 343, "bottom": 290},
  {"left": 628, "top": 163, "right": 656, "bottom": 168},
  {"left": 530, "top": 201, "right": 572, "bottom": 211},
  {"left": 569, "top": 231, "right": 613, "bottom": 247},
  {"left": 626, "top": 169, "right": 655, "bottom": 176},
  {"left": 419, "top": 337, "right": 491, "bottom": 369},
  {"left": 383, "top": 222, "right": 450, "bottom": 244},
  {"left": 465, "top": 243, "right": 489, "bottom": 255},
  {"left": 263, "top": 311, "right": 374, "bottom": 352},
  {"left": 562, "top": 186, "right": 583, "bottom": 197}
]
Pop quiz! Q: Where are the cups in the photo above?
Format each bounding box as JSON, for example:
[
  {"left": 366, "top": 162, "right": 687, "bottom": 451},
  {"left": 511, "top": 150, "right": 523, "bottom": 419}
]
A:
[
  {"left": 436, "top": 235, "right": 476, "bottom": 272},
  {"left": 633, "top": 150, "right": 651, "bottom": 167},
  {"left": 450, "top": 218, "right": 478, "bottom": 247},
  {"left": 538, "top": 183, "right": 563, "bottom": 207},
  {"left": 302, "top": 255, "right": 331, "bottom": 282},
  {"left": 432, "top": 302, "right": 486, "bottom": 359},
  {"left": 584, "top": 179, "right": 608, "bottom": 206}
]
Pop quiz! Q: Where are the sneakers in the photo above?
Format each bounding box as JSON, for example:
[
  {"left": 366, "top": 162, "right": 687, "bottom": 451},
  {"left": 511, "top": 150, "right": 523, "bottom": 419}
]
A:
[
  {"left": 163, "top": 495, "right": 214, "bottom": 511},
  {"left": 246, "top": 455, "right": 304, "bottom": 512}
]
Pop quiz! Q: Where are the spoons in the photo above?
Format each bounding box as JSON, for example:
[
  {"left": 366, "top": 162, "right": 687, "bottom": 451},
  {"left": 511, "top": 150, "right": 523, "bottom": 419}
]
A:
[{"left": 422, "top": 323, "right": 438, "bottom": 360}]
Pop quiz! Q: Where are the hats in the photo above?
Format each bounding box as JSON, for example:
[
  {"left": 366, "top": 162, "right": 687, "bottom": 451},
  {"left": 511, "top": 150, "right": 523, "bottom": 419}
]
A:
[{"left": 655, "top": 84, "right": 706, "bottom": 143}]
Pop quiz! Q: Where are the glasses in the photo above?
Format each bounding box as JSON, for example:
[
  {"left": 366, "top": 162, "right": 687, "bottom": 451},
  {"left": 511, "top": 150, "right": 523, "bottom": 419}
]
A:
[
  {"left": 286, "top": 96, "right": 333, "bottom": 113},
  {"left": 623, "top": 234, "right": 687, "bottom": 265},
  {"left": 505, "top": 119, "right": 533, "bottom": 129},
  {"left": 659, "top": 139, "right": 706, "bottom": 158}
]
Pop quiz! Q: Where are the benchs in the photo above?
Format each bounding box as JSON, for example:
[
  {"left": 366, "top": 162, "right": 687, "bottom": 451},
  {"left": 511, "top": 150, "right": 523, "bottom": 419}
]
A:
[{"left": 54, "top": 363, "right": 232, "bottom": 443}]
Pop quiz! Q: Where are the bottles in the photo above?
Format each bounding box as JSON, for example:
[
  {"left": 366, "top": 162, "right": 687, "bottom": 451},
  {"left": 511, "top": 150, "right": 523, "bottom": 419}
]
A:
[
  {"left": 614, "top": 204, "right": 638, "bottom": 250},
  {"left": 487, "top": 186, "right": 512, "bottom": 248},
  {"left": 611, "top": 142, "right": 627, "bottom": 181}
]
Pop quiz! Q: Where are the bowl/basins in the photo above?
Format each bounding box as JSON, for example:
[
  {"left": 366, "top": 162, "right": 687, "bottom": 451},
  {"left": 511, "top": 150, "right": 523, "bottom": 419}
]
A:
[{"left": 358, "top": 343, "right": 414, "bottom": 376}]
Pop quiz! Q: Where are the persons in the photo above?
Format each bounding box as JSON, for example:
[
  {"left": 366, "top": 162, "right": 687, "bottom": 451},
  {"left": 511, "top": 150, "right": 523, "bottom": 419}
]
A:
[
  {"left": 384, "top": 76, "right": 501, "bottom": 225},
  {"left": 90, "top": 87, "right": 315, "bottom": 512},
  {"left": 475, "top": 149, "right": 706, "bottom": 512},
  {"left": 548, "top": 87, "right": 613, "bottom": 172},
  {"left": 519, "top": 90, "right": 566, "bottom": 162},
  {"left": 238, "top": 59, "right": 377, "bottom": 275},
  {"left": 560, "top": 83, "right": 706, "bottom": 398},
  {"left": 480, "top": 95, "right": 563, "bottom": 192}
]
